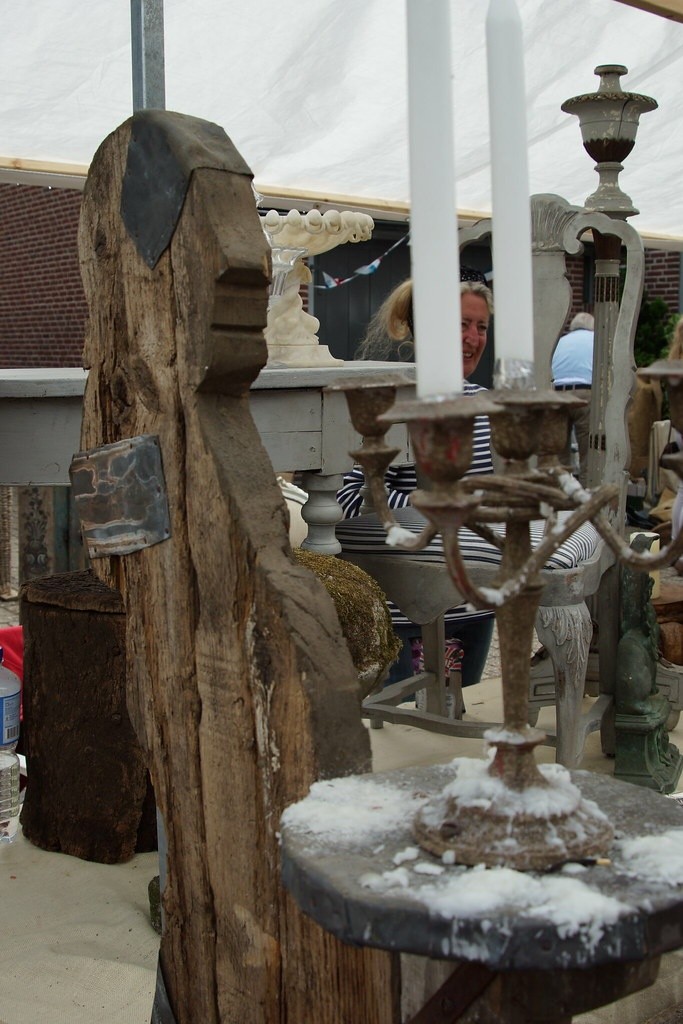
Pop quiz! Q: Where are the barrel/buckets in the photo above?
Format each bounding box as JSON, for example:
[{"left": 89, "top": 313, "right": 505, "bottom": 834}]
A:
[{"left": 0, "top": 626, "right": 23, "bottom": 680}]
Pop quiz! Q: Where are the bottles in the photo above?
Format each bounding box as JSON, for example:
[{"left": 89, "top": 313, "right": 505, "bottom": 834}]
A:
[
  {"left": 0, "top": 751, "right": 21, "bottom": 846},
  {"left": 0, "top": 646, "right": 22, "bottom": 753}
]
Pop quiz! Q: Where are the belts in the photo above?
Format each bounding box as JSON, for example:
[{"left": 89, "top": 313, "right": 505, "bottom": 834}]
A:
[{"left": 554, "top": 383, "right": 591, "bottom": 392}]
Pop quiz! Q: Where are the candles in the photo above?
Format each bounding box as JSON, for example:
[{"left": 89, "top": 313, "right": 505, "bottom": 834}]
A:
[
  {"left": 405, "top": 0, "right": 466, "bottom": 401},
  {"left": 485, "top": 0, "right": 537, "bottom": 365}
]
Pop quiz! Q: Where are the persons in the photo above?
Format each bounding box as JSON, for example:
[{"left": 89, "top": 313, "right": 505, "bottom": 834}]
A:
[
  {"left": 550, "top": 312, "right": 596, "bottom": 489},
  {"left": 335, "top": 265, "right": 493, "bottom": 702}
]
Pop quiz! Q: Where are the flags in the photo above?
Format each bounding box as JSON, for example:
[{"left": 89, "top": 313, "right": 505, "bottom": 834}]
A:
[{"left": 322, "top": 258, "right": 381, "bottom": 289}]
